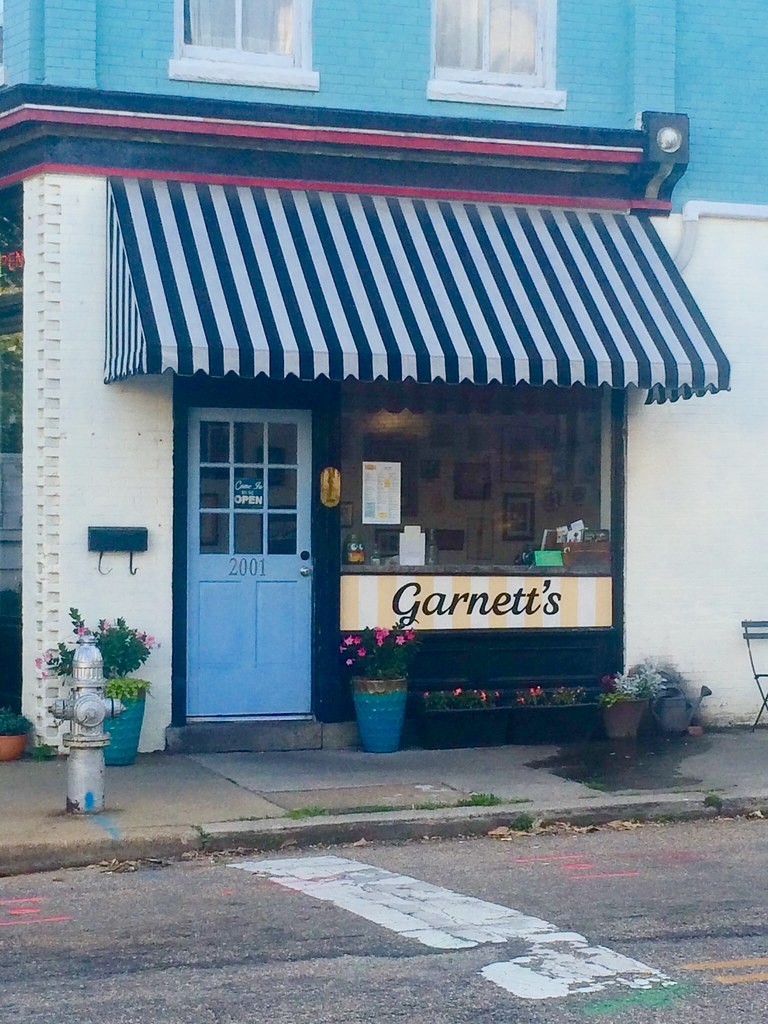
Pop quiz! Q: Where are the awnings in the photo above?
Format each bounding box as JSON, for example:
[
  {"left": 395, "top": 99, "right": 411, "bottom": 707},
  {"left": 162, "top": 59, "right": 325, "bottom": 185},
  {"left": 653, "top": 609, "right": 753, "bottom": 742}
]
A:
[{"left": 104, "top": 175, "right": 733, "bottom": 404}]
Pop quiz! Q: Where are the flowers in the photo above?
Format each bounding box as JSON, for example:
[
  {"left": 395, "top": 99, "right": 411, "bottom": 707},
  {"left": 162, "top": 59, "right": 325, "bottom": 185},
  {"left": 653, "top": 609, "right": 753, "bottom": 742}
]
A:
[
  {"left": 421, "top": 685, "right": 587, "bottom": 710},
  {"left": 35, "top": 607, "right": 161, "bottom": 701},
  {"left": 597, "top": 665, "right": 668, "bottom": 708},
  {"left": 340, "top": 623, "right": 423, "bottom": 680}
]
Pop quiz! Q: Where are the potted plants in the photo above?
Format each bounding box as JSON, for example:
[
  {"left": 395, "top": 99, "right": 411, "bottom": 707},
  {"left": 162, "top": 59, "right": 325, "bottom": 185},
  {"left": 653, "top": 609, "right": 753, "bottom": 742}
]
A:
[{"left": 0, "top": 705, "right": 34, "bottom": 761}]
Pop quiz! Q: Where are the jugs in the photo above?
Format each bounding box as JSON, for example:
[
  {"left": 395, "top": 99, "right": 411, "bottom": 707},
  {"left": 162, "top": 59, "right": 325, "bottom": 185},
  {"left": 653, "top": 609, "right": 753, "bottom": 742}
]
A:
[{"left": 650, "top": 686, "right": 712, "bottom": 734}]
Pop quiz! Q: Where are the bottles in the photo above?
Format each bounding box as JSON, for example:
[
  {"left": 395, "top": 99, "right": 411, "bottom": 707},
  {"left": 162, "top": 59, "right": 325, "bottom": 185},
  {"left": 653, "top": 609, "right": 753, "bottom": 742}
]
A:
[
  {"left": 371, "top": 544, "right": 380, "bottom": 565},
  {"left": 425, "top": 528, "right": 439, "bottom": 565}
]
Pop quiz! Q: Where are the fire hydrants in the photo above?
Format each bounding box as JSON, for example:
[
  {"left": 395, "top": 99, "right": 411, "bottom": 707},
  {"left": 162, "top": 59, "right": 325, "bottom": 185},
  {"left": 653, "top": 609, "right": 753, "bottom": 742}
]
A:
[{"left": 46, "top": 633, "right": 127, "bottom": 814}]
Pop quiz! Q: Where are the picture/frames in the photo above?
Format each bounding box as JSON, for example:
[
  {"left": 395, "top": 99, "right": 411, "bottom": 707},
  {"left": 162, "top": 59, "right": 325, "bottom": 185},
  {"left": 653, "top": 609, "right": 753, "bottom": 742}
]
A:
[
  {"left": 375, "top": 529, "right": 403, "bottom": 557},
  {"left": 453, "top": 462, "right": 491, "bottom": 500},
  {"left": 503, "top": 492, "right": 535, "bottom": 541},
  {"left": 501, "top": 424, "right": 539, "bottom": 484}
]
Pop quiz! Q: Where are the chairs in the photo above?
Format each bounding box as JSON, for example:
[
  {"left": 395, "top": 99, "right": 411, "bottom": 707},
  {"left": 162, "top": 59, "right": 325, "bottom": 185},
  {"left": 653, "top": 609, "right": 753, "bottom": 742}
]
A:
[{"left": 741, "top": 619, "right": 768, "bottom": 734}]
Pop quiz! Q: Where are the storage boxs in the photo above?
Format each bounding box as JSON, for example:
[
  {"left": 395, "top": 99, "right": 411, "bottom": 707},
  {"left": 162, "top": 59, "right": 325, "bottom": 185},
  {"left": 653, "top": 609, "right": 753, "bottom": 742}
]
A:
[{"left": 556, "top": 542, "right": 611, "bottom": 567}]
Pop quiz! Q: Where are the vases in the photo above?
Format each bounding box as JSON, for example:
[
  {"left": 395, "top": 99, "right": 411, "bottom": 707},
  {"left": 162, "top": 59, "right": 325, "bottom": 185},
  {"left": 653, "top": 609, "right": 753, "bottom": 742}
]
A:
[
  {"left": 103, "top": 689, "right": 146, "bottom": 766},
  {"left": 417, "top": 703, "right": 601, "bottom": 750},
  {"left": 350, "top": 677, "right": 409, "bottom": 753},
  {"left": 603, "top": 697, "right": 650, "bottom": 742}
]
